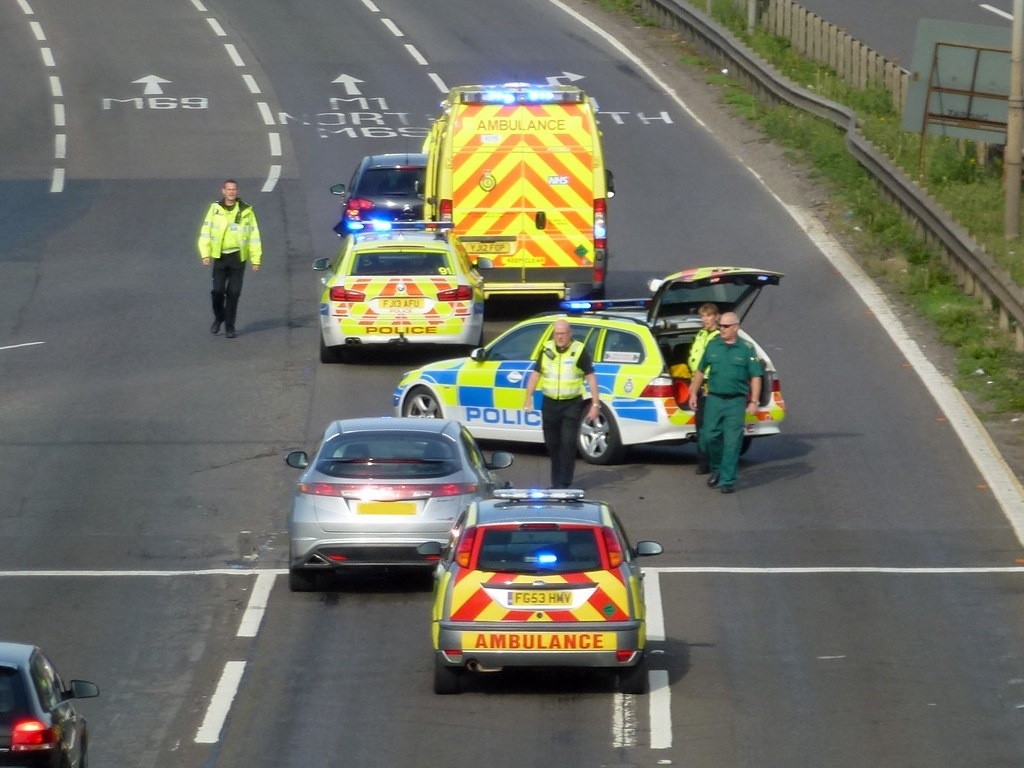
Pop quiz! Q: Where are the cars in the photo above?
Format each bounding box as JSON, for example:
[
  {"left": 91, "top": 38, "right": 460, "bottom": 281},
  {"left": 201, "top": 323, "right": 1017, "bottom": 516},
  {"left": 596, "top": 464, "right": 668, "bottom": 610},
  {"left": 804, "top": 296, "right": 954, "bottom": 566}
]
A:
[
  {"left": 284, "top": 413, "right": 515, "bottom": 591},
  {"left": 330, "top": 154, "right": 428, "bottom": 234},
  {"left": 313, "top": 219, "right": 484, "bottom": 366},
  {"left": 1, "top": 644, "right": 100, "bottom": 768}
]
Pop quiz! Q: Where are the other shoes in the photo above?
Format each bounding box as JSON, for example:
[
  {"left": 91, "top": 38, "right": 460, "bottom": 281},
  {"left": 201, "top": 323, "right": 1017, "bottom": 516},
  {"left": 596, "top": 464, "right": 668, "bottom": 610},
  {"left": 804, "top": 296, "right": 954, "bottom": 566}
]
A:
[
  {"left": 721, "top": 486, "right": 730, "bottom": 493},
  {"left": 211, "top": 319, "right": 222, "bottom": 333},
  {"left": 707, "top": 475, "right": 719, "bottom": 487},
  {"left": 696, "top": 464, "right": 708, "bottom": 475},
  {"left": 225, "top": 328, "right": 235, "bottom": 338}
]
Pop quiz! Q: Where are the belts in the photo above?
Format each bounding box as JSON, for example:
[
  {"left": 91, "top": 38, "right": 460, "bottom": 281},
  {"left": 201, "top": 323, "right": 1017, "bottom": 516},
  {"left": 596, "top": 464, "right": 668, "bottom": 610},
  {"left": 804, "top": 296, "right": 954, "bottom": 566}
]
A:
[
  {"left": 708, "top": 391, "right": 737, "bottom": 399},
  {"left": 545, "top": 396, "right": 573, "bottom": 403}
]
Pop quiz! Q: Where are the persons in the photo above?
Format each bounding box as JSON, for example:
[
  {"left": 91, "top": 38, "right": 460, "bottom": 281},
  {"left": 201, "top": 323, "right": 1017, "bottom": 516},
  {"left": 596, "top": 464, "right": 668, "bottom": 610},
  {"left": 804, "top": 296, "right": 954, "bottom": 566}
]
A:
[
  {"left": 197, "top": 179, "right": 263, "bottom": 338},
  {"left": 523, "top": 321, "right": 601, "bottom": 490},
  {"left": 687, "top": 303, "right": 764, "bottom": 493}
]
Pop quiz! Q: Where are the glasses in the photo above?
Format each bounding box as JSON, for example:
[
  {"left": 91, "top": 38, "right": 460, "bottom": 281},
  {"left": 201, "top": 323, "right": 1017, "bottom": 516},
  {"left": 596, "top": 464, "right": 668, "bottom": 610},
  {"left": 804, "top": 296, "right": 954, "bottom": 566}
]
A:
[{"left": 718, "top": 323, "right": 739, "bottom": 329}]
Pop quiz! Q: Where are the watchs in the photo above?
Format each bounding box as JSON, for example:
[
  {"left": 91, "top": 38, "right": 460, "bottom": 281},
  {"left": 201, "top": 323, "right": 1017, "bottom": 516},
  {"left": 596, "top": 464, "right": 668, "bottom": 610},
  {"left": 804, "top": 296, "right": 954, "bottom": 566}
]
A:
[{"left": 750, "top": 398, "right": 761, "bottom": 407}]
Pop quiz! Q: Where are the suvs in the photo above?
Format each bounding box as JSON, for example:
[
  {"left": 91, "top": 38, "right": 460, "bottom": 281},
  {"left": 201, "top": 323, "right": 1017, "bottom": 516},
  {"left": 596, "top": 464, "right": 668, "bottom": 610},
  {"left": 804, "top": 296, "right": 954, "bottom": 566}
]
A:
[{"left": 392, "top": 266, "right": 787, "bottom": 464}]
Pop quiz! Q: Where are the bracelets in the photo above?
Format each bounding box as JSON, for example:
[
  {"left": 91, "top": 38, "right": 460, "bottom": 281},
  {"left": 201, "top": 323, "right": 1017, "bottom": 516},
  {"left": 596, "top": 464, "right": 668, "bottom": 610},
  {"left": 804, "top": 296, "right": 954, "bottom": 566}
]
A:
[{"left": 592, "top": 403, "right": 601, "bottom": 408}]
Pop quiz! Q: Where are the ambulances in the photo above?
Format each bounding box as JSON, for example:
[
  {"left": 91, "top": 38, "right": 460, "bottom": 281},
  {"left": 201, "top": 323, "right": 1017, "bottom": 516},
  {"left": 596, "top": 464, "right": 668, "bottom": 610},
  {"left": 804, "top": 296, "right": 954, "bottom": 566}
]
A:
[
  {"left": 413, "top": 488, "right": 665, "bottom": 694},
  {"left": 416, "top": 86, "right": 616, "bottom": 309}
]
